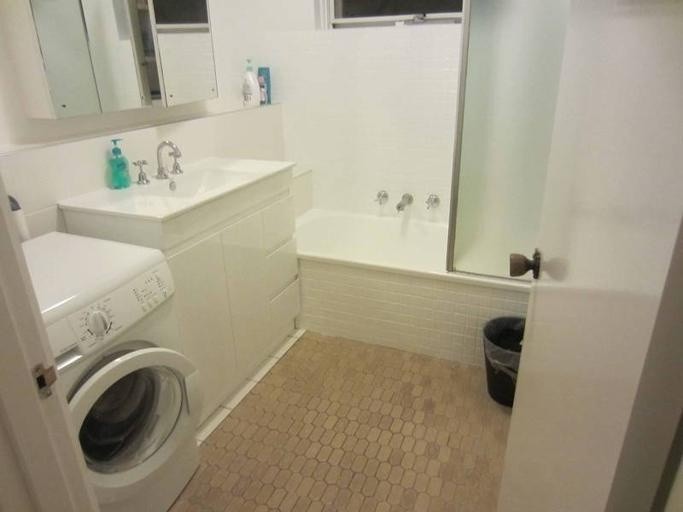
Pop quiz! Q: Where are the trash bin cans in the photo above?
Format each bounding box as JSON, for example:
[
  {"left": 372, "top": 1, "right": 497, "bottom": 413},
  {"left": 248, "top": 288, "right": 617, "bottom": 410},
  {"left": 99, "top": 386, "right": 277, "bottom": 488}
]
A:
[{"left": 482, "top": 317, "right": 526, "bottom": 407}]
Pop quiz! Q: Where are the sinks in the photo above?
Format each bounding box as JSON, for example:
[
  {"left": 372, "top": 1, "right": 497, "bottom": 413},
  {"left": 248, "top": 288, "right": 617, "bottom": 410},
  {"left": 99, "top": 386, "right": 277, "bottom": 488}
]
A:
[{"left": 136, "top": 168, "right": 247, "bottom": 207}]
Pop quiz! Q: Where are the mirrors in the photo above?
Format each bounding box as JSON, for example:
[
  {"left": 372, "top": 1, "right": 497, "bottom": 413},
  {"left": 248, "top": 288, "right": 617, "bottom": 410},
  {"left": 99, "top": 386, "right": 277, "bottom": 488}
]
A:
[{"left": 27, "top": 1, "right": 219, "bottom": 117}]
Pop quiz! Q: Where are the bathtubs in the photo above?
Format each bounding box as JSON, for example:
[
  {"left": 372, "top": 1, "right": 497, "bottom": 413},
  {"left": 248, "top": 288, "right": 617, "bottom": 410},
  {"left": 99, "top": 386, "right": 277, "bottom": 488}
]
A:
[{"left": 294, "top": 211, "right": 533, "bottom": 294}]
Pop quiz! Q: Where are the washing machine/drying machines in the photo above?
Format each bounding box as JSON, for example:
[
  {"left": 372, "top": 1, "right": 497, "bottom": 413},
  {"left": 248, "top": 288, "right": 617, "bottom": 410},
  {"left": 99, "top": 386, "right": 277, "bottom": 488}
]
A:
[{"left": 21, "top": 231, "right": 204, "bottom": 512}]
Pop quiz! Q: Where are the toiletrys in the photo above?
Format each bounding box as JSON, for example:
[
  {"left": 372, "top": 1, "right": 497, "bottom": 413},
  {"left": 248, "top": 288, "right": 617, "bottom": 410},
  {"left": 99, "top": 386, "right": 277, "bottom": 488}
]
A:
[
  {"left": 108, "top": 138, "right": 130, "bottom": 190},
  {"left": 242, "top": 59, "right": 261, "bottom": 106},
  {"left": 258, "top": 68, "right": 272, "bottom": 103}
]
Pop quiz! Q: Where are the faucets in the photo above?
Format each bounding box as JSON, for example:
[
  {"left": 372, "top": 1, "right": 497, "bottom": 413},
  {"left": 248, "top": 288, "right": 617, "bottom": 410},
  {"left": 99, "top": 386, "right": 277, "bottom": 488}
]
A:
[
  {"left": 397, "top": 193, "right": 413, "bottom": 211},
  {"left": 156, "top": 141, "right": 182, "bottom": 170}
]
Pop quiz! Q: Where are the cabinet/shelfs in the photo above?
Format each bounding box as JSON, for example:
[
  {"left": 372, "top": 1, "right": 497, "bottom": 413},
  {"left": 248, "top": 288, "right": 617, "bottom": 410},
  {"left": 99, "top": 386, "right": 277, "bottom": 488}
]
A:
[
  {"left": 9, "top": 0, "right": 224, "bottom": 123},
  {"left": 62, "top": 169, "right": 298, "bottom": 430}
]
